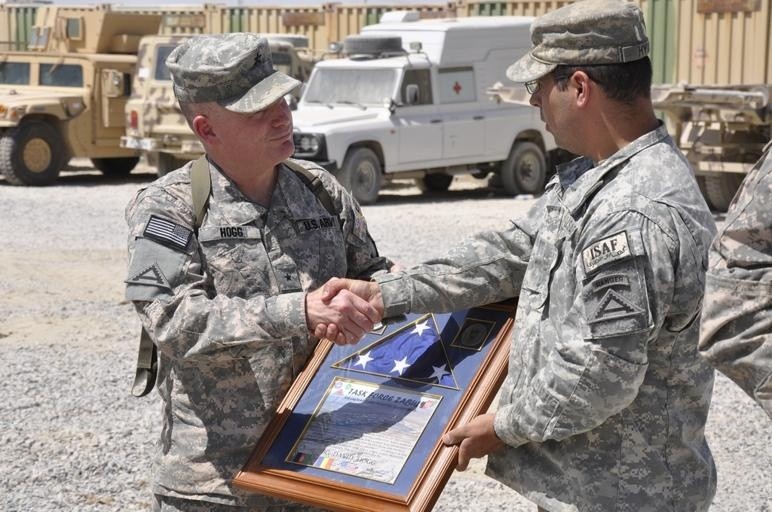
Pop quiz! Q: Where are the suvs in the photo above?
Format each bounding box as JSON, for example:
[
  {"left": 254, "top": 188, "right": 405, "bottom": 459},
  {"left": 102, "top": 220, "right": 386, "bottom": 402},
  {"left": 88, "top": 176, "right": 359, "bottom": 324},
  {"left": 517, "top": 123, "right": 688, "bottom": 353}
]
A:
[{"left": 120, "top": 33, "right": 344, "bottom": 183}]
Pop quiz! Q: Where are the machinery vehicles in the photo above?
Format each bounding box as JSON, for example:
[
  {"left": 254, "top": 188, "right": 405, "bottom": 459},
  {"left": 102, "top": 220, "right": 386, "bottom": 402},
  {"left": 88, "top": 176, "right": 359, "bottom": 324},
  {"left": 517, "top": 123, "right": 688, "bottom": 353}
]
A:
[{"left": 0, "top": 4, "right": 165, "bottom": 183}]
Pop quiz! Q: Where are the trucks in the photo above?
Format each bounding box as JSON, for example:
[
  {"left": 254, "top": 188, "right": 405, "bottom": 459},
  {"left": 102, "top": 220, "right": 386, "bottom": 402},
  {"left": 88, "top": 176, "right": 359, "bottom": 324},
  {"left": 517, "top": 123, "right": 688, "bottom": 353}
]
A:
[
  {"left": 625, "top": 1, "right": 772, "bottom": 212},
  {"left": 289, "top": 10, "right": 563, "bottom": 206}
]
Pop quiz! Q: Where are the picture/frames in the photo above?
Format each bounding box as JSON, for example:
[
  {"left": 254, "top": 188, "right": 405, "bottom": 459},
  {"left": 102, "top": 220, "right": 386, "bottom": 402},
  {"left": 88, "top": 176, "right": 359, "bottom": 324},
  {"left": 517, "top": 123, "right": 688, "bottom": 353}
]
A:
[{"left": 232, "top": 302, "right": 516, "bottom": 512}]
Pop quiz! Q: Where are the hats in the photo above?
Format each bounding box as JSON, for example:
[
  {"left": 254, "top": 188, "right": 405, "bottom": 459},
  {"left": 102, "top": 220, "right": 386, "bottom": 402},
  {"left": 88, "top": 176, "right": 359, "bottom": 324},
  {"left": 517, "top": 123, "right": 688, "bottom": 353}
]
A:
[
  {"left": 166, "top": 31, "right": 303, "bottom": 115},
  {"left": 506, "top": 1, "right": 649, "bottom": 82}
]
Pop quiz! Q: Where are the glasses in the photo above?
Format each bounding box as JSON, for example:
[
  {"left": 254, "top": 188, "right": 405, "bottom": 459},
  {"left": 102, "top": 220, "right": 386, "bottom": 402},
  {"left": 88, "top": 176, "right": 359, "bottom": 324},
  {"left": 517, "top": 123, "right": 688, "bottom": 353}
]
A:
[{"left": 525, "top": 75, "right": 572, "bottom": 94}]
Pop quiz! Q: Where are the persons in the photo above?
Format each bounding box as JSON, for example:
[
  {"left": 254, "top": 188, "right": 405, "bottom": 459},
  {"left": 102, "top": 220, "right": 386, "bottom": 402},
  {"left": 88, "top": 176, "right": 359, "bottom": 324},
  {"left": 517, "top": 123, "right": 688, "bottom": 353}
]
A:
[
  {"left": 698, "top": 138, "right": 771, "bottom": 419},
  {"left": 125, "top": 32, "right": 395, "bottom": 512},
  {"left": 313, "top": 1, "right": 716, "bottom": 512}
]
